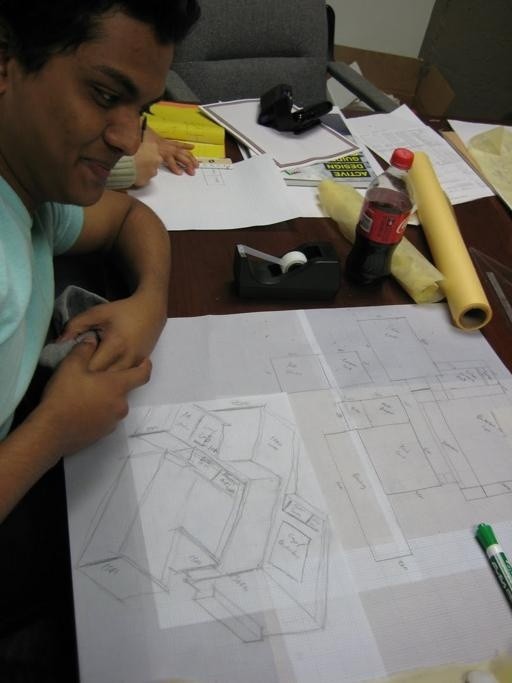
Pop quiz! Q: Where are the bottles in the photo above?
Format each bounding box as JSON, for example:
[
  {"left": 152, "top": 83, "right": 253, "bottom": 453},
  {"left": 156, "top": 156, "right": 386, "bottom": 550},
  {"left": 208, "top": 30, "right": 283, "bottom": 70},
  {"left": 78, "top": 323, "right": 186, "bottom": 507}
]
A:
[{"left": 338, "top": 147, "right": 419, "bottom": 292}]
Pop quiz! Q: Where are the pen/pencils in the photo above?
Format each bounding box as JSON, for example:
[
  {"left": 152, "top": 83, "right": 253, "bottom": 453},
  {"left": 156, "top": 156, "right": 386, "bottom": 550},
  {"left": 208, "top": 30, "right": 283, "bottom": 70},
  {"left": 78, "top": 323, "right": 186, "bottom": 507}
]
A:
[{"left": 476, "top": 522, "right": 512, "bottom": 605}]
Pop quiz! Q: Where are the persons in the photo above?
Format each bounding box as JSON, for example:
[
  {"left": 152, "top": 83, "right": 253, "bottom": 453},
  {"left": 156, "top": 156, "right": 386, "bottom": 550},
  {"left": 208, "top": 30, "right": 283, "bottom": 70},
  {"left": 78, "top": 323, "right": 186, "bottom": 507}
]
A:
[
  {"left": 104, "top": 122, "right": 197, "bottom": 188},
  {"left": 1, "top": 2, "right": 198, "bottom": 529}
]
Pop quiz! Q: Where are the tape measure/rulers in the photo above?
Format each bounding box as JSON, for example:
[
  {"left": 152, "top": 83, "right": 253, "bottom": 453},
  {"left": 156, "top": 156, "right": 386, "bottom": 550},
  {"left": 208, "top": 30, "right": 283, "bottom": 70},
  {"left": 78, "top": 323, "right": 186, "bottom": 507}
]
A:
[{"left": 469, "top": 247, "right": 512, "bottom": 333}]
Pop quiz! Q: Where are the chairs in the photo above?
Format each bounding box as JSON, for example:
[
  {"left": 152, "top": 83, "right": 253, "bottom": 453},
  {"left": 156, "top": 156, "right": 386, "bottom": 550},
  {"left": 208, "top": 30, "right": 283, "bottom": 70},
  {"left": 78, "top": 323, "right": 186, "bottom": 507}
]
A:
[{"left": 167, "top": 0, "right": 401, "bottom": 112}]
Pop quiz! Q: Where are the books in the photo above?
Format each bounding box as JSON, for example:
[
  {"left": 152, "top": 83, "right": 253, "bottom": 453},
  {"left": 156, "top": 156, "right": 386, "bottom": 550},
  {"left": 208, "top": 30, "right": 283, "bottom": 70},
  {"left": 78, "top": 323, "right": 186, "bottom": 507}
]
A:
[
  {"left": 246, "top": 102, "right": 380, "bottom": 191},
  {"left": 199, "top": 94, "right": 362, "bottom": 172}
]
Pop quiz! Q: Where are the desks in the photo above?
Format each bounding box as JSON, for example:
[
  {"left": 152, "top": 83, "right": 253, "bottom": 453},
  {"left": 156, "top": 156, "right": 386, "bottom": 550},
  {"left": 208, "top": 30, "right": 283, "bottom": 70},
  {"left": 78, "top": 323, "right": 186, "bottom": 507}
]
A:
[{"left": 123, "top": 107, "right": 512, "bottom": 682}]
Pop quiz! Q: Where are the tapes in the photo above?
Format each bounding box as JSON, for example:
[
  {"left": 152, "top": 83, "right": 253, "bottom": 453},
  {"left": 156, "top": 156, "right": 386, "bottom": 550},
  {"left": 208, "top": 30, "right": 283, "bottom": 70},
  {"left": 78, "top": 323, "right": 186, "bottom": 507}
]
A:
[{"left": 238, "top": 244, "right": 307, "bottom": 274}]
